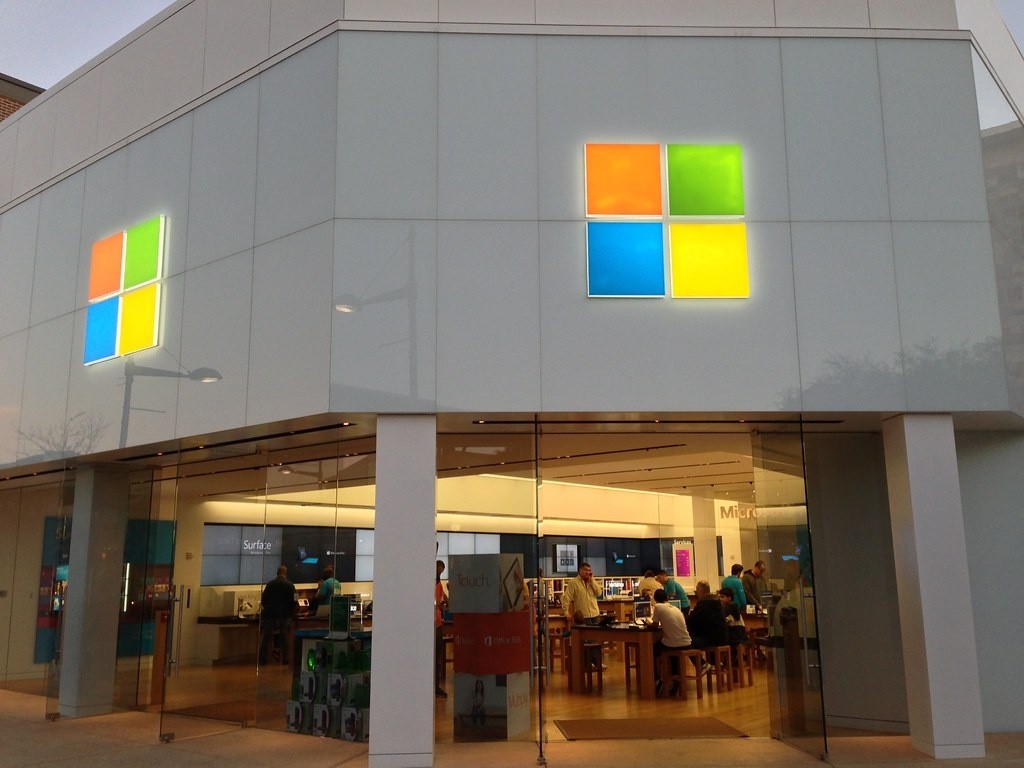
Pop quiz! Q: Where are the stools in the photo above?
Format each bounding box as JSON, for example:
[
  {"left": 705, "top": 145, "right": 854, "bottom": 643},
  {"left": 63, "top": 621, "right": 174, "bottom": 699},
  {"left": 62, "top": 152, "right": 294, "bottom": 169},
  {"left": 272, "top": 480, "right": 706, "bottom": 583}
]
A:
[{"left": 443, "top": 615, "right": 772, "bottom": 699}]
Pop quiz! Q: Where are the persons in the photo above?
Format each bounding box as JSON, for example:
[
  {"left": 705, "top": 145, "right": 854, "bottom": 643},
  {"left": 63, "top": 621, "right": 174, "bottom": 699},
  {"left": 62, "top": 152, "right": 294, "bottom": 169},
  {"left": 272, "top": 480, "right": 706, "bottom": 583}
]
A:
[
  {"left": 435, "top": 560, "right": 449, "bottom": 698},
  {"left": 308, "top": 567, "right": 341, "bottom": 616},
  {"left": 562, "top": 563, "right": 608, "bottom": 671},
  {"left": 470, "top": 679, "right": 489, "bottom": 736},
  {"left": 259, "top": 565, "right": 295, "bottom": 666},
  {"left": 637, "top": 560, "right": 770, "bottom": 699}
]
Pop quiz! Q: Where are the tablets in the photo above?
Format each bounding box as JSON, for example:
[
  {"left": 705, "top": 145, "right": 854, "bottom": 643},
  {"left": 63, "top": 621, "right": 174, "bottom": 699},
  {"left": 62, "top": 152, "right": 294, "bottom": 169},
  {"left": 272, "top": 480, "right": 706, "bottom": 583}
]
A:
[{"left": 503, "top": 558, "right": 524, "bottom": 608}]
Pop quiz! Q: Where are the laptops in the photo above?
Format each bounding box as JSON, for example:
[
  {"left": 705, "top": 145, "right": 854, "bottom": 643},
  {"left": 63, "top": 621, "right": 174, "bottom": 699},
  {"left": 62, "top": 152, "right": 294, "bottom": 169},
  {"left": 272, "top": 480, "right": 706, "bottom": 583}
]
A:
[
  {"left": 668, "top": 601, "right": 681, "bottom": 611},
  {"left": 635, "top": 602, "right": 654, "bottom": 624}
]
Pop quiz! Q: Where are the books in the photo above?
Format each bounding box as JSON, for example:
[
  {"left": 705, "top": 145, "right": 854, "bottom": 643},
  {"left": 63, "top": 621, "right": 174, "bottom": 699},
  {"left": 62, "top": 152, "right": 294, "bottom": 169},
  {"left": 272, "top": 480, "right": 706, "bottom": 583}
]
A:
[
  {"left": 546, "top": 580, "right": 574, "bottom": 606},
  {"left": 342, "top": 592, "right": 372, "bottom": 632},
  {"left": 604, "top": 579, "right": 640, "bottom": 599},
  {"left": 629, "top": 625, "right": 646, "bottom": 629}
]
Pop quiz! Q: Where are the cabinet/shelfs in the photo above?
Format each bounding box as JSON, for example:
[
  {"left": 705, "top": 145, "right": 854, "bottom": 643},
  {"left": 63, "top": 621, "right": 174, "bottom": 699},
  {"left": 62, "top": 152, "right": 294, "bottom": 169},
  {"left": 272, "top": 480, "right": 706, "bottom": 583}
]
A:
[{"left": 523, "top": 576, "right": 642, "bottom": 601}]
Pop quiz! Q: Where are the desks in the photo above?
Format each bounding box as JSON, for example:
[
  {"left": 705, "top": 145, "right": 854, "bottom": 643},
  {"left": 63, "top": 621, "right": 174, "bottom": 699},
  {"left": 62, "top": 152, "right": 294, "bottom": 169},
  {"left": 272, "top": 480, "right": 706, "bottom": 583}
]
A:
[
  {"left": 535, "top": 605, "right": 564, "bottom": 614},
  {"left": 287, "top": 616, "right": 373, "bottom": 669},
  {"left": 596, "top": 599, "right": 636, "bottom": 662}
]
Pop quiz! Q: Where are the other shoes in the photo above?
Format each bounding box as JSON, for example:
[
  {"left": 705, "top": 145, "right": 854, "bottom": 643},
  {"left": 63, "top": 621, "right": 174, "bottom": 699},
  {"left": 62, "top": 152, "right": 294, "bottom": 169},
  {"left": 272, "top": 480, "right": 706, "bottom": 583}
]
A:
[
  {"left": 435, "top": 687, "right": 447, "bottom": 697},
  {"left": 656, "top": 680, "right": 664, "bottom": 695},
  {"left": 259, "top": 660, "right": 267, "bottom": 665},
  {"left": 591, "top": 663, "right": 608, "bottom": 671},
  {"left": 668, "top": 681, "right": 677, "bottom": 696},
  {"left": 282, "top": 660, "right": 289, "bottom": 665}
]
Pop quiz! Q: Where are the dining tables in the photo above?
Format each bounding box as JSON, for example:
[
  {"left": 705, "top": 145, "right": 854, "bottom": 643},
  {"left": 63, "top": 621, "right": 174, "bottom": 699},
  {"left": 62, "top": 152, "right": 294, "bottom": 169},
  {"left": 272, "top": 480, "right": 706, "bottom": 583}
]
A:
[
  {"left": 742, "top": 613, "right": 769, "bottom": 669},
  {"left": 570, "top": 623, "right": 661, "bottom": 697},
  {"left": 535, "top": 613, "right": 571, "bottom": 669}
]
